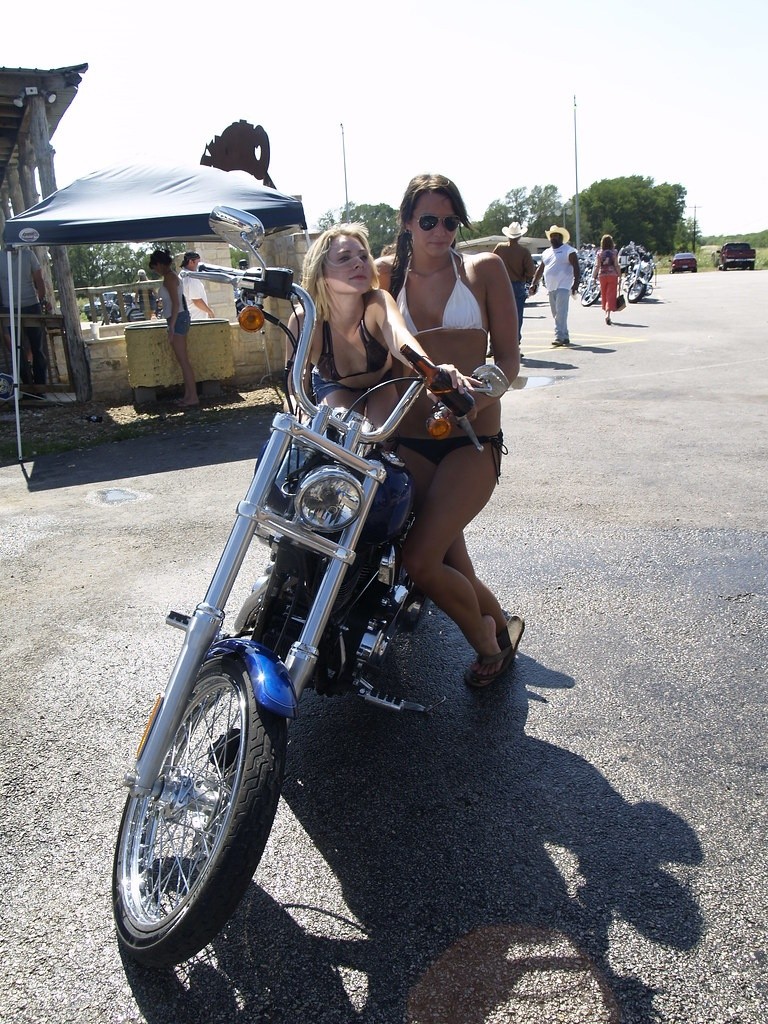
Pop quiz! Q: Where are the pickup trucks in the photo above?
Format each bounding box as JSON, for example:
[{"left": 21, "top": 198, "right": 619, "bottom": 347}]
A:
[{"left": 716, "top": 242, "right": 756, "bottom": 271}]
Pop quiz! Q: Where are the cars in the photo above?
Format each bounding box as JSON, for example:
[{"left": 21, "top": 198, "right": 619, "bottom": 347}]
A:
[{"left": 668, "top": 253, "right": 697, "bottom": 273}]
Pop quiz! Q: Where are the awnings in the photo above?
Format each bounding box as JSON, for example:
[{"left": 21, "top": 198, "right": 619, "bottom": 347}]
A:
[{"left": 3, "top": 157, "right": 315, "bottom": 462}]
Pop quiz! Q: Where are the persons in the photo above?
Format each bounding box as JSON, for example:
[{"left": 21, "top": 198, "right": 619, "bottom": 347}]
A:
[
  {"left": 485, "top": 222, "right": 622, "bottom": 359},
  {"left": 284, "top": 221, "right": 486, "bottom": 436},
  {"left": 374, "top": 173, "right": 525, "bottom": 689},
  {"left": 0, "top": 229, "right": 54, "bottom": 399},
  {"left": 138, "top": 249, "right": 214, "bottom": 408}
]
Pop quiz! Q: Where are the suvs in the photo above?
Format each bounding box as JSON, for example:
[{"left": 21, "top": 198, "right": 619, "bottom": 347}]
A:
[{"left": 83, "top": 290, "right": 119, "bottom": 321}]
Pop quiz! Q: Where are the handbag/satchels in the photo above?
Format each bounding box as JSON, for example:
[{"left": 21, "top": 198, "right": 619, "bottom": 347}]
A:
[{"left": 616, "top": 295, "right": 629, "bottom": 314}]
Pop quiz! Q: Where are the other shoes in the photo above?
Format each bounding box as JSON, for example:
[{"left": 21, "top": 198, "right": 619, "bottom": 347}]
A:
[
  {"left": 552, "top": 338, "right": 565, "bottom": 346},
  {"left": 605, "top": 316, "right": 612, "bottom": 325},
  {"left": 562, "top": 339, "right": 572, "bottom": 344},
  {"left": 25, "top": 391, "right": 47, "bottom": 401}
]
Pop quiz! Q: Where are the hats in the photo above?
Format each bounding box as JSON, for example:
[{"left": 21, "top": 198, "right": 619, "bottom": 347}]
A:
[
  {"left": 544, "top": 224, "right": 570, "bottom": 244},
  {"left": 137, "top": 269, "right": 147, "bottom": 276},
  {"left": 184, "top": 251, "right": 201, "bottom": 260},
  {"left": 501, "top": 221, "right": 529, "bottom": 240}
]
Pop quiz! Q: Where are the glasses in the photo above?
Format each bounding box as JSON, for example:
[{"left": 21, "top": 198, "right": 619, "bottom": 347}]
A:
[{"left": 418, "top": 212, "right": 460, "bottom": 233}]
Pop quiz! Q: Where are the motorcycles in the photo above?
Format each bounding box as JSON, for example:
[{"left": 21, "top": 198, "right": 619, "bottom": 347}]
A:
[
  {"left": 108, "top": 208, "right": 449, "bottom": 970},
  {"left": 573, "top": 241, "right": 657, "bottom": 307},
  {"left": 100, "top": 293, "right": 164, "bottom": 326}
]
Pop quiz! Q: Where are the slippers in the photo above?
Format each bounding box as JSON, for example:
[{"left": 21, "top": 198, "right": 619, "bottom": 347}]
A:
[
  {"left": 172, "top": 397, "right": 200, "bottom": 409},
  {"left": 463, "top": 613, "right": 527, "bottom": 688}
]
